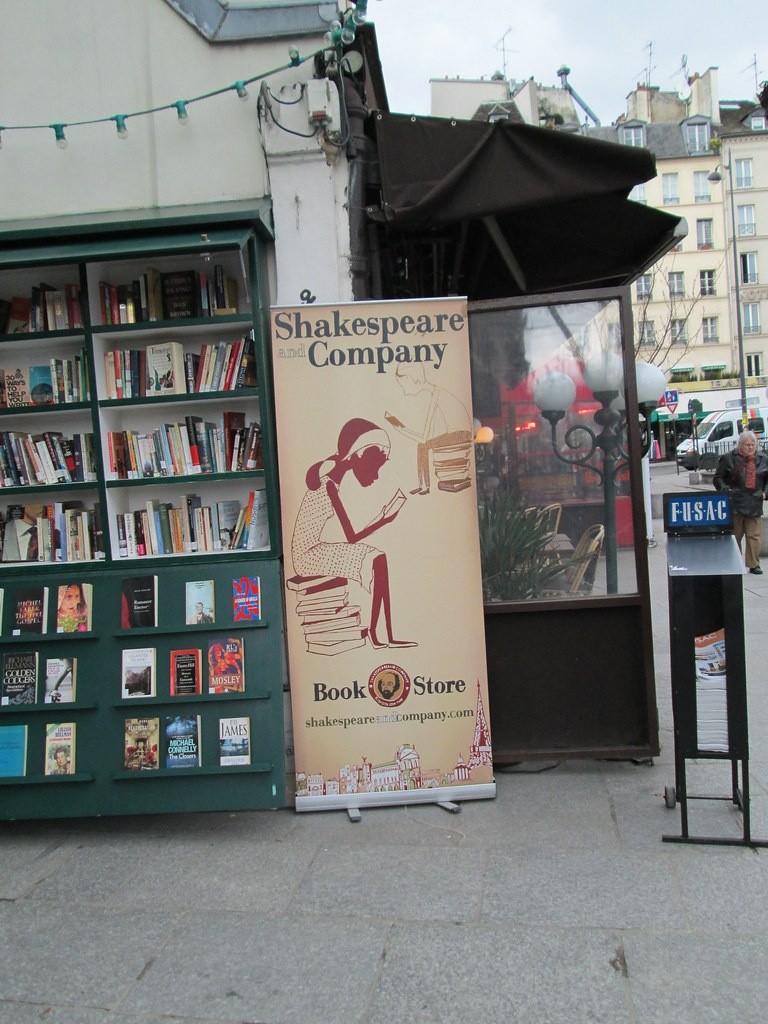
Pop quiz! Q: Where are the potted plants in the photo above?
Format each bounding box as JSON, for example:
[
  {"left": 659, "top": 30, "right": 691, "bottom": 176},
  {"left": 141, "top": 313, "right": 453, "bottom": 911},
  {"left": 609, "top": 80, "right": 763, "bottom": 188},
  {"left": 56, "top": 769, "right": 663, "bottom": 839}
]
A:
[{"left": 708, "top": 138, "right": 722, "bottom": 154}]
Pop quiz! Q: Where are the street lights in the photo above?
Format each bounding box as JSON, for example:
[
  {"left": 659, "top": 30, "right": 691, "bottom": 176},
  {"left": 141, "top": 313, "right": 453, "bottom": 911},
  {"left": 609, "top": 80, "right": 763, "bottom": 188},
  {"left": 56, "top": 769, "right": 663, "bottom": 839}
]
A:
[
  {"left": 707, "top": 147, "right": 749, "bottom": 434},
  {"left": 532, "top": 349, "right": 667, "bottom": 595}
]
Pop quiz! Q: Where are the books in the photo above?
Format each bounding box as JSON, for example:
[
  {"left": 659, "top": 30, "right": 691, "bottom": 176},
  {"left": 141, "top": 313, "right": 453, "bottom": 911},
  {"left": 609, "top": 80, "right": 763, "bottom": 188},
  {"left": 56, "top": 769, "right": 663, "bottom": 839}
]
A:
[
  {"left": 0, "top": 431, "right": 97, "bottom": 488},
  {"left": 1, "top": 582, "right": 93, "bottom": 777},
  {"left": 116, "top": 489, "right": 269, "bottom": 558},
  {"left": 107, "top": 411, "right": 263, "bottom": 479},
  {"left": 103, "top": 329, "right": 257, "bottom": 400},
  {"left": 100, "top": 264, "right": 238, "bottom": 325},
  {"left": 0, "top": 347, "right": 90, "bottom": 408},
  {"left": 121, "top": 575, "right": 262, "bottom": 771},
  {"left": 0, "top": 500, "right": 105, "bottom": 561},
  {"left": 0, "top": 281, "right": 85, "bottom": 335}
]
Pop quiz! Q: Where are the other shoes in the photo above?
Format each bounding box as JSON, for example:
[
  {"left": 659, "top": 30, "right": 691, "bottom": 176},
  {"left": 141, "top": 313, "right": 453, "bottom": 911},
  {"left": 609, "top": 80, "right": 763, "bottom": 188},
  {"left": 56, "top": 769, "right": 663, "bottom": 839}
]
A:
[{"left": 749, "top": 565, "right": 763, "bottom": 574}]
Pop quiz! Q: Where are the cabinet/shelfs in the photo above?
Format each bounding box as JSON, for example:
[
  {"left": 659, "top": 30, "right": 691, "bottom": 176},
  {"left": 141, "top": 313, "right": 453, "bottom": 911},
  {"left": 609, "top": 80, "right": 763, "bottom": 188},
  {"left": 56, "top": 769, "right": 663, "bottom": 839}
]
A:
[
  {"left": 0, "top": 560, "right": 286, "bottom": 819},
  {"left": 0, "top": 229, "right": 283, "bottom": 576}
]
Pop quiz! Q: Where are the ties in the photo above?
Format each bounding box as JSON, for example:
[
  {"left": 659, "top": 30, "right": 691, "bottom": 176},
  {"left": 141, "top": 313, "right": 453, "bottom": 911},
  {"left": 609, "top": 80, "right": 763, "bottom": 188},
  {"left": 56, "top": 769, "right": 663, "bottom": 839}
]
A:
[{"left": 726, "top": 450, "right": 757, "bottom": 489}]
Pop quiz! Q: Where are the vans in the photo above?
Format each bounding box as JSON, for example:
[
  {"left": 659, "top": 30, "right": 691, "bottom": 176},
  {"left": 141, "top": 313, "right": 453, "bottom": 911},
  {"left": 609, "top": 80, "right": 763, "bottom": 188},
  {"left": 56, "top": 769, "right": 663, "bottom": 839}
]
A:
[{"left": 675, "top": 407, "right": 768, "bottom": 470}]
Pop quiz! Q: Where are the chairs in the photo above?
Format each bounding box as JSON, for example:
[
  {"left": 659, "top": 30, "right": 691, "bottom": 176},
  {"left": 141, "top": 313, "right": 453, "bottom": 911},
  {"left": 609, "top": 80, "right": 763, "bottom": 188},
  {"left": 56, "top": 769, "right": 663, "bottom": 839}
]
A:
[
  {"left": 525, "top": 503, "right": 562, "bottom": 568},
  {"left": 540, "top": 524, "right": 605, "bottom": 597}
]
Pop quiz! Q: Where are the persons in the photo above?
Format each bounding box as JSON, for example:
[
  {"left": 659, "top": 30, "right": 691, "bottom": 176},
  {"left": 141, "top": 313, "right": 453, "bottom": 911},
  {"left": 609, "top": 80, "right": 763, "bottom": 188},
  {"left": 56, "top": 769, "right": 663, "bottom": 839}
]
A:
[{"left": 713, "top": 430, "right": 768, "bottom": 574}]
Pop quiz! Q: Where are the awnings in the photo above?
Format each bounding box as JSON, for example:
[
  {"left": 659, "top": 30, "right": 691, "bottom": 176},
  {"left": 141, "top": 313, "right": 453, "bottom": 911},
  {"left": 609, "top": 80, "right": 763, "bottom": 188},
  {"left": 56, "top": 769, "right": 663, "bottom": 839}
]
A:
[{"left": 368, "top": 110, "right": 689, "bottom": 293}]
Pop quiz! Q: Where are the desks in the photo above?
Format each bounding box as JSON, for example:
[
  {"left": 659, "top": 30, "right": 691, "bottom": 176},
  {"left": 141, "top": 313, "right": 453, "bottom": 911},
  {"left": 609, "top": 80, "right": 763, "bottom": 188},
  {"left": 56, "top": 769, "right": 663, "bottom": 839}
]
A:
[{"left": 539, "top": 533, "right": 575, "bottom": 554}]
